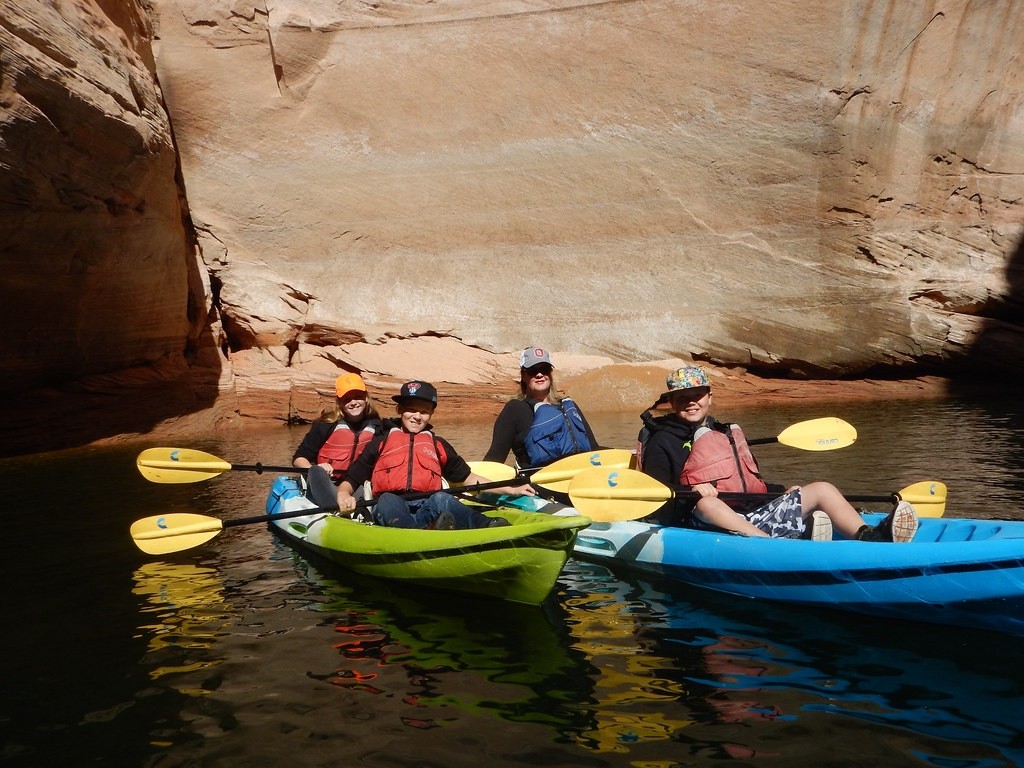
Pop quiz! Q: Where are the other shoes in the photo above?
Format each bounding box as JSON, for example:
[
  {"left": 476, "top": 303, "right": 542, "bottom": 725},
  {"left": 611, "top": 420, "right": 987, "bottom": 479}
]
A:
[
  {"left": 426, "top": 512, "right": 456, "bottom": 530},
  {"left": 489, "top": 515, "right": 512, "bottom": 528}
]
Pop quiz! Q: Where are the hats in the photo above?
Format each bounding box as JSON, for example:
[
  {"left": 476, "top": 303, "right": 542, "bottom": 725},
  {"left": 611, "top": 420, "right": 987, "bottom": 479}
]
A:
[
  {"left": 664, "top": 366, "right": 711, "bottom": 396},
  {"left": 520, "top": 347, "right": 555, "bottom": 369},
  {"left": 392, "top": 381, "right": 437, "bottom": 409},
  {"left": 334, "top": 373, "right": 367, "bottom": 399}
]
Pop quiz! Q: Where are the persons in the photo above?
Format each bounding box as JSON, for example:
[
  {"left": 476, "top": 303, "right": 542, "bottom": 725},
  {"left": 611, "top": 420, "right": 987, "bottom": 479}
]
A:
[
  {"left": 292, "top": 373, "right": 382, "bottom": 521},
  {"left": 338, "top": 382, "right": 535, "bottom": 530},
  {"left": 642, "top": 366, "right": 918, "bottom": 542},
  {"left": 482, "top": 348, "right": 615, "bottom": 477}
]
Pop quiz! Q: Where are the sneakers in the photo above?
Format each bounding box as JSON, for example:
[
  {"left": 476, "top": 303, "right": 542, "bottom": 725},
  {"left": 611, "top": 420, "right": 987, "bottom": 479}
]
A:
[
  {"left": 801, "top": 510, "right": 833, "bottom": 541},
  {"left": 872, "top": 500, "right": 917, "bottom": 544}
]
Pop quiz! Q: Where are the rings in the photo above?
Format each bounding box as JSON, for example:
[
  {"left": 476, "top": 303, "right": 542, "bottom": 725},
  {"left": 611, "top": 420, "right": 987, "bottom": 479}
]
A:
[{"left": 347, "top": 505, "right": 351, "bottom": 508}]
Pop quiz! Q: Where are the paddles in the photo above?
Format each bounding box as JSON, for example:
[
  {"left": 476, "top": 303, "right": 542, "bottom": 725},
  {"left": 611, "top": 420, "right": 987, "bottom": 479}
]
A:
[
  {"left": 567, "top": 467, "right": 949, "bottom": 523},
  {"left": 136, "top": 446, "right": 352, "bottom": 485},
  {"left": 463, "top": 416, "right": 857, "bottom": 483},
  {"left": 129, "top": 448, "right": 637, "bottom": 555}
]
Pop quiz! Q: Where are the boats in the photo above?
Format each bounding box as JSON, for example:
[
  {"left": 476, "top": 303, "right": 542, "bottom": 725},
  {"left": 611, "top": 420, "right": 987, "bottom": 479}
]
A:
[
  {"left": 265, "top": 446, "right": 592, "bottom": 607},
  {"left": 476, "top": 457, "right": 1023, "bottom": 639}
]
